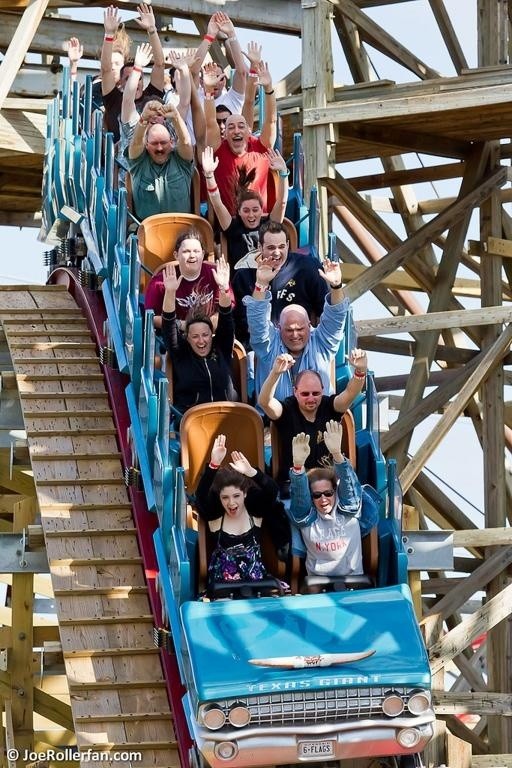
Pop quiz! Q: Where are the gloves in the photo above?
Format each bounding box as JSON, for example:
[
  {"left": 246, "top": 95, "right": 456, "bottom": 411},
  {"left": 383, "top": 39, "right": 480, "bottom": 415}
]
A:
[
  {"left": 292, "top": 432, "right": 311, "bottom": 465},
  {"left": 324, "top": 419, "right": 342, "bottom": 453}
]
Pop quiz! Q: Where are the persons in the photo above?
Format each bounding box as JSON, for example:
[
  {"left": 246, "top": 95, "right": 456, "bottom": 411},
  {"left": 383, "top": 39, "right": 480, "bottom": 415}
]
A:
[{"left": 55, "top": 1, "right": 382, "bottom": 600}]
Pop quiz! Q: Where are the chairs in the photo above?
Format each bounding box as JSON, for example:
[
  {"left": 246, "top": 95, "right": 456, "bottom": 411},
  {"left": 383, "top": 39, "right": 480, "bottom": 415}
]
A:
[{"left": 90, "top": 106, "right": 378, "bottom": 592}]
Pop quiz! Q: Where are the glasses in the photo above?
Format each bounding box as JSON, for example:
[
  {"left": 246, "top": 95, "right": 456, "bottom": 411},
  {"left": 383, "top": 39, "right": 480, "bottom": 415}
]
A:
[
  {"left": 312, "top": 490, "right": 336, "bottom": 499},
  {"left": 301, "top": 391, "right": 322, "bottom": 396},
  {"left": 217, "top": 118, "right": 227, "bottom": 124}
]
